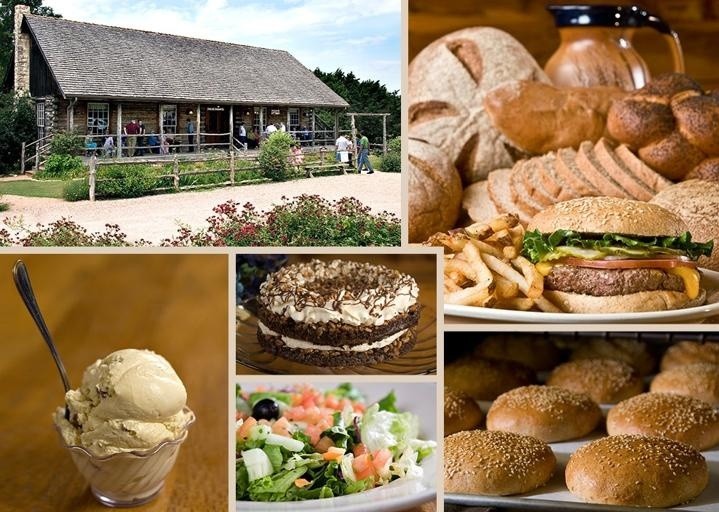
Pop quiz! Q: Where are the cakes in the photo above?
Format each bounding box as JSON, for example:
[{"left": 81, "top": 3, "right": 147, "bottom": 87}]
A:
[{"left": 257, "top": 258, "right": 420, "bottom": 367}]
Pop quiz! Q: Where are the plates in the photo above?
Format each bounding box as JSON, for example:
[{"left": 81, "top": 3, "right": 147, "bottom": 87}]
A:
[
  {"left": 444, "top": 237, "right": 719, "bottom": 323},
  {"left": 235, "top": 277, "right": 436, "bottom": 375},
  {"left": 237, "top": 430, "right": 436, "bottom": 512}
]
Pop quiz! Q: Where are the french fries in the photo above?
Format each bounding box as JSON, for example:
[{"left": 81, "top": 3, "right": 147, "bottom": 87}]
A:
[{"left": 421, "top": 213, "right": 561, "bottom": 313}]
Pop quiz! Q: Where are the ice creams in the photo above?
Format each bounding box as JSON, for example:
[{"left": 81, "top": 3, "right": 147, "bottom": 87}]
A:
[{"left": 53, "top": 349, "right": 191, "bottom": 501}]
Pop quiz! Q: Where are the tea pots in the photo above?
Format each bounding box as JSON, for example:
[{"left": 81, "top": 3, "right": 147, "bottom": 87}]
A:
[{"left": 538, "top": 5, "right": 687, "bottom": 91}]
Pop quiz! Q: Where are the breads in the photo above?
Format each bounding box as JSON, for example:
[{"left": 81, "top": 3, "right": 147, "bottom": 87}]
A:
[
  {"left": 443, "top": 334, "right": 719, "bottom": 508},
  {"left": 408, "top": 27, "right": 719, "bottom": 270}
]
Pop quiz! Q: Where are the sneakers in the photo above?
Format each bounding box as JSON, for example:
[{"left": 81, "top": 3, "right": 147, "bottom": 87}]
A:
[{"left": 358, "top": 168, "right": 374, "bottom": 175}]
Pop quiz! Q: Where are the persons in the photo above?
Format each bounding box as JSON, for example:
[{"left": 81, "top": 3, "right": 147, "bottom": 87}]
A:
[{"left": 83, "top": 116, "right": 374, "bottom": 175}]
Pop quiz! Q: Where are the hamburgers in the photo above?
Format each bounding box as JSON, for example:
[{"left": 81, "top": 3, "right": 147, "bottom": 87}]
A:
[{"left": 520, "top": 197, "right": 713, "bottom": 314}]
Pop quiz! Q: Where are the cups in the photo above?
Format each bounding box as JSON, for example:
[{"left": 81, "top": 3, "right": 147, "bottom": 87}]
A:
[{"left": 54, "top": 405, "right": 198, "bottom": 509}]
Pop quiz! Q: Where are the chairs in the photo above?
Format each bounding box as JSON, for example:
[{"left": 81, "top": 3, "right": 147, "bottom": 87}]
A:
[
  {"left": 244, "top": 127, "right": 336, "bottom": 150},
  {"left": 80, "top": 129, "right": 185, "bottom": 159}
]
